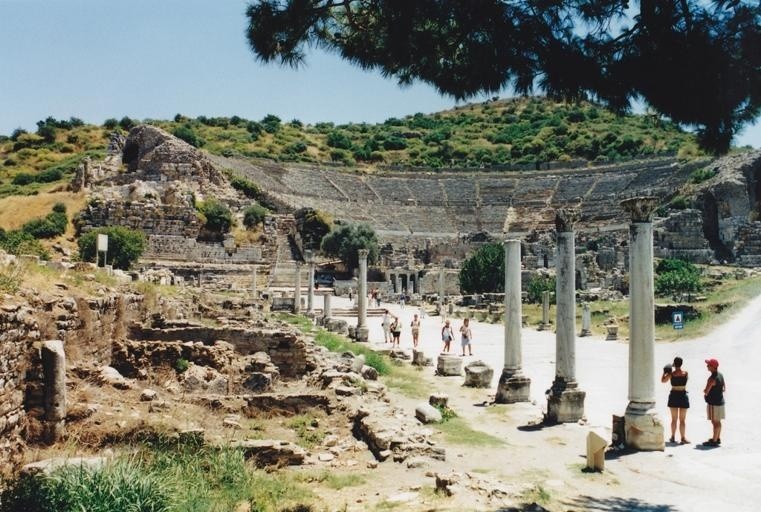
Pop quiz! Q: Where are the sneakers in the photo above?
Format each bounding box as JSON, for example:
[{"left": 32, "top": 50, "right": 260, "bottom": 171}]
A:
[{"left": 702, "top": 439, "right": 720, "bottom": 446}]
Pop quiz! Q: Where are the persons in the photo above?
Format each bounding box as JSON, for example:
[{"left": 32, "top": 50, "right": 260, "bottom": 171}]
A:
[
  {"left": 460, "top": 318, "right": 472, "bottom": 356},
  {"left": 382, "top": 311, "right": 402, "bottom": 348},
  {"left": 442, "top": 320, "right": 455, "bottom": 352},
  {"left": 315, "top": 280, "right": 319, "bottom": 289},
  {"left": 400, "top": 293, "right": 406, "bottom": 309},
  {"left": 348, "top": 288, "right": 381, "bottom": 308},
  {"left": 411, "top": 314, "right": 420, "bottom": 347},
  {"left": 703, "top": 359, "right": 725, "bottom": 446},
  {"left": 662, "top": 357, "right": 690, "bottom": 444}
]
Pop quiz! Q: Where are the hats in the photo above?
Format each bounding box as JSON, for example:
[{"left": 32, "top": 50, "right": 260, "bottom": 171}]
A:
[
  {"left": 705, "top": 359, "right": 719, "bottom": 368},
  {"left": 674, "top": 358, "right": 682, "bottom": 364}
]
